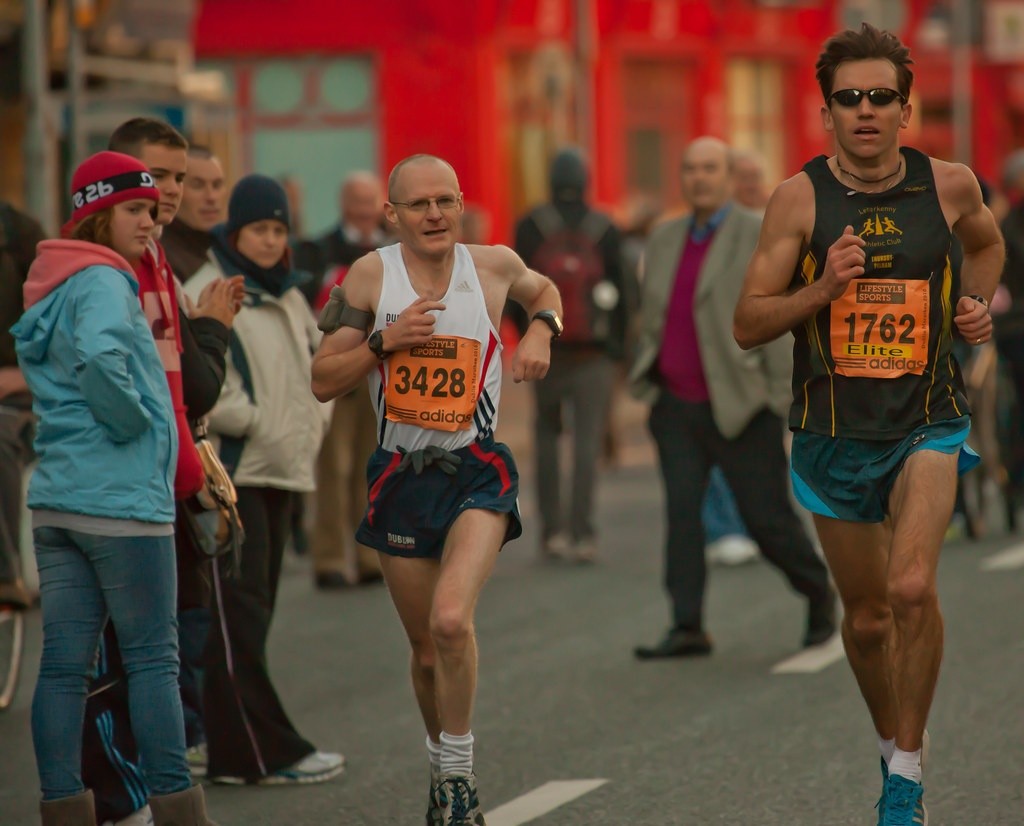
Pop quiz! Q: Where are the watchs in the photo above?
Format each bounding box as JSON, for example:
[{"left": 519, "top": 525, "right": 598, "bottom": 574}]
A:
[
  {"left": 533, "top": 310, "right": 563, "bottom": 340},
  {"left": 369, "top": 330, "right": 391, "bottom": 360},
  {"left": 970, "top": 294, "right": 989, "bottom": 311}
]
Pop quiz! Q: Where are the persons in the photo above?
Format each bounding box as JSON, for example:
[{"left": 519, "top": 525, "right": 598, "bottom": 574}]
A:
[
  {"left": 620, "top": 137, "right": 835, "bottom": 660},
  {"left": 0, "top": 118, "right": 390, "bottom": 826},
  {"left": 734, "top": 22, "right": 1007, "bottom": 825},
  {"left": 944, "top": 175, "right": 1024, "bottom": 532},
  {"left": 310, "top": 153, "right": 564, "bottom": 825},
  {"left": 506, "top": 151, "right": 635, "bottom": 560}
]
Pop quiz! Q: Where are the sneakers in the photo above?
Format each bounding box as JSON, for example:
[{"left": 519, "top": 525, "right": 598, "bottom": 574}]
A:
[
  {"left": 426, "top": 785, "right": 444, "bottom": 826},
  {"left": 873, "top": 729, "right": 928, "bottom": 826},
  {"left": 435, "top": 771, "right": 487, "bottom": 826}
]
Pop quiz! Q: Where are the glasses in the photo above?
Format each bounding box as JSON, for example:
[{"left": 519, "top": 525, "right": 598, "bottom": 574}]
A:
[
  {"left": 390, "top": 194, "right": 458, "bottom": 213},
  {"left": 826, "top": 87, "right": 907, "bottom": 111}
]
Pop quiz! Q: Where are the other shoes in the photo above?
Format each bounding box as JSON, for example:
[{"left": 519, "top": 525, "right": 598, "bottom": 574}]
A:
[
  {"left": 573, "top": 533, "right": 604, "bottom": 565},
  {"left": 313, "top": 569, "right": 349, "bottom": 591},
  {"left": 354, "top": 567, "right": 384, "bottom": 587},
  {"left": 802, "top": 586, "right": 836, "bottom": 645},
  {"left": 705, "top": 534, "right": 761, "bottom": 565},
  {"left": 545, "top": 533, "right": 574, "bottom": 561},
  {"left": 184, "top": 741, "right": 209, "bottom": 777},
  {"left": 291, "top": 523, "right": 310, "bottom": 555},
  {"left": 635, "top": 629, "right": 710, "bottom": 658},
  {"left": 0, "top": 576, "right": 29, "bottom": 612},
  {"left": 212, "top": 751, "right": 345, "bottom": 786}
]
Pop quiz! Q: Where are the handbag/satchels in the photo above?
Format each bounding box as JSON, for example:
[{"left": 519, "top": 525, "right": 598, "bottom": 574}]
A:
[{"left": 188, "top": 417, "right": 248, "bottom": 555}]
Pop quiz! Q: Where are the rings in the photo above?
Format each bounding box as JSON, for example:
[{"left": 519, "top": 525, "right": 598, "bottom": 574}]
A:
[{"left": 976, "top": 338, "right": 983, "bottom": 345}]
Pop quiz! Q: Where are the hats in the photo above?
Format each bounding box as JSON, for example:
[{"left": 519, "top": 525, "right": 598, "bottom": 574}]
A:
[
  {"left": 69, "top": 151, "right": 160, "bottom": 222},
  {"left": 224, "top": 175, "right": 292, "bottom": 237}
]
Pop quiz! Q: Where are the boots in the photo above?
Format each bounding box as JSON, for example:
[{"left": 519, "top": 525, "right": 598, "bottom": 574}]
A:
[
  {"left": 148, "top": 783, "right": 210, "bottom": 826},
  {"left": 39, "top": 789, "right": 97, "bottom": 826}
]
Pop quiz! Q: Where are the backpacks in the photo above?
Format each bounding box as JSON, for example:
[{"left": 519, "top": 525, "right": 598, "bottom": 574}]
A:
[{"left": 527, "top": 201, "right": 613, "bottom": 345}]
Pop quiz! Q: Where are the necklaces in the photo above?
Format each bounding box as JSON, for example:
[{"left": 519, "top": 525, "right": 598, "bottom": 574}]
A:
[{"left": 837, "top": 159, "right": 902, "bottom": 183}]
